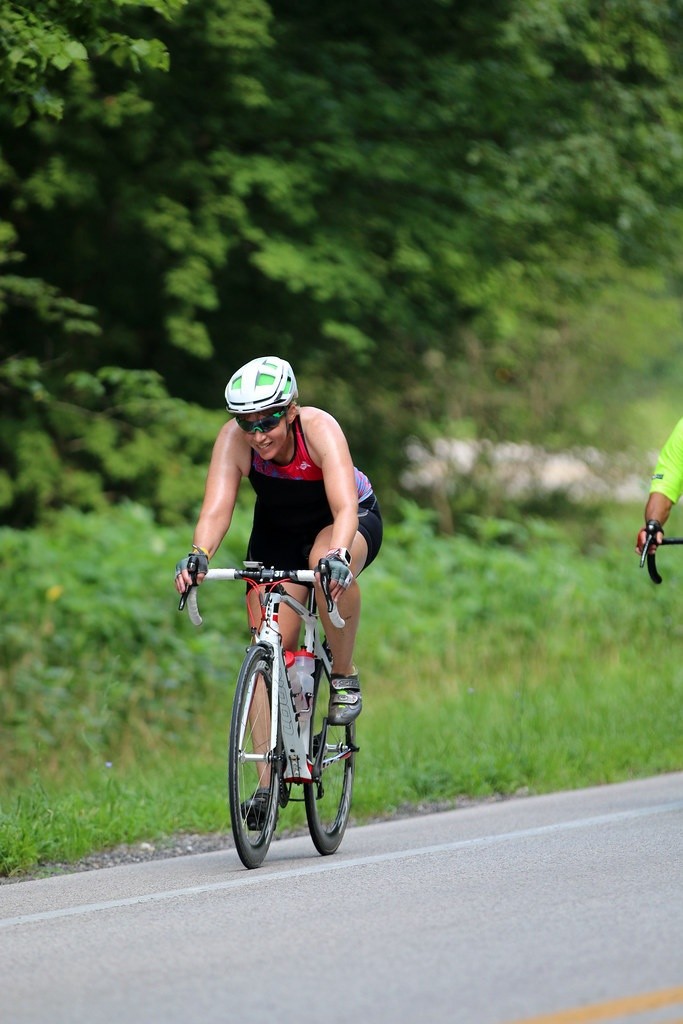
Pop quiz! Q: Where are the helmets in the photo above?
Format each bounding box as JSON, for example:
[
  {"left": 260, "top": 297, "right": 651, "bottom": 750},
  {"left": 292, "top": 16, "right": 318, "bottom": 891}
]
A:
[{"left": 224, "top": 356, "right": 299, "bottom": 415}]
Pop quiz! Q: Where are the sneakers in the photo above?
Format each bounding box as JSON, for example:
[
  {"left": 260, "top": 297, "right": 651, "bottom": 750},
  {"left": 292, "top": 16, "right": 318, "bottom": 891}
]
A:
[
  {"left": 327, "top": 661, "right": 364, "bottom": 726},
  {"left": 241, "top": 788, "right": 279, "bottom": 820}
]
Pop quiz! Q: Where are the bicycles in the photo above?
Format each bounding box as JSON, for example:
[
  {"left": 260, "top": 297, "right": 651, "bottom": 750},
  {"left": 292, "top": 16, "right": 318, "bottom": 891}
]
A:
[{"left": 179, "top": 553, "right": 361, "bottom": 870}]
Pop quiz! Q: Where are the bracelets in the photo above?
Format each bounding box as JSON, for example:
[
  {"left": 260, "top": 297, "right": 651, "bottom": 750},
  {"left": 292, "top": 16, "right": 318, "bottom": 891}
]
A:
[{"left": 190, "top": 547, "right": 212, "bottom": 560}]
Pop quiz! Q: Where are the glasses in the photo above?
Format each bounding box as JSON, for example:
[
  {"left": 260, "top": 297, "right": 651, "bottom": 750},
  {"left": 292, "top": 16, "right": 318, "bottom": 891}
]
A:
[{"left": 235, "top": 408, "right": 289, "bottom": 435}]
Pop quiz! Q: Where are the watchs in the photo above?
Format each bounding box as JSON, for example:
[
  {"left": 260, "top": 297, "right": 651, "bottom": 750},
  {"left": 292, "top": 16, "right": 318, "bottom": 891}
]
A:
[{"left": 324, "top": 546, "right": 353, "bottom": 566}]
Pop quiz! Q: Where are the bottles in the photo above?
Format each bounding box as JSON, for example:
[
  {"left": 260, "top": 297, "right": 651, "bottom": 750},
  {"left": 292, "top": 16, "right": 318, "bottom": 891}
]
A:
[
  {"left": 284, "top": 651, "right": 304, "bottom": 721},
  {"left": 296, "top": 650, "right": 317, "bottom": 716}
]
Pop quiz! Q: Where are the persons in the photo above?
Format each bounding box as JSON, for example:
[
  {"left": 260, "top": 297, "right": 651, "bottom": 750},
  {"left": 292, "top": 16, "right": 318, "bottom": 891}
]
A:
[
  {"left": 635, "top": 418, "right": 683, "bottom": 555},
  {"left": 175, "top": 355, "right": 384, "bottom": 824}
]
